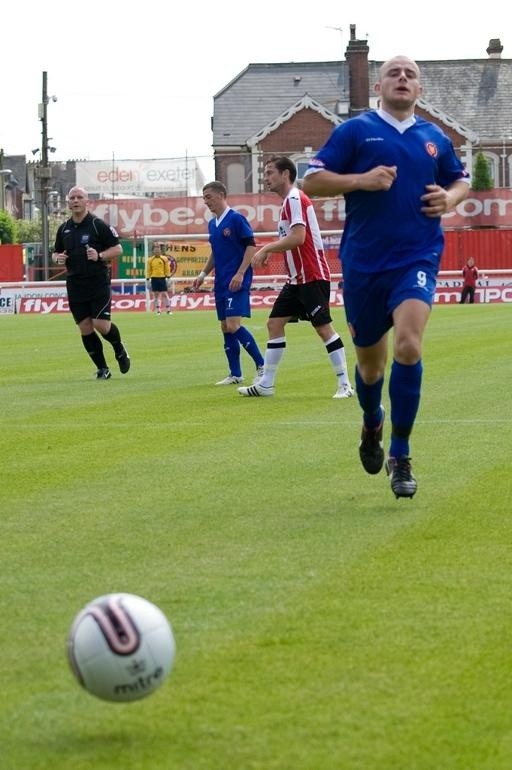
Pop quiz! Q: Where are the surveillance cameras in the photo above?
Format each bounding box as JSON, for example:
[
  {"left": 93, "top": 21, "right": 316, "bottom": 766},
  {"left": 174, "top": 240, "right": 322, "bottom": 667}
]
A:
[{"left": 53, "top": 94, "right": 57, "bottom": 102}]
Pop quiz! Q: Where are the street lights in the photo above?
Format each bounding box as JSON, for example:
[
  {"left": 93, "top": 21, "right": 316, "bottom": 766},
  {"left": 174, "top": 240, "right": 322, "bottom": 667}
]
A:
[{"left": 0, "top": 169, "right": 12, "bottom": 177}]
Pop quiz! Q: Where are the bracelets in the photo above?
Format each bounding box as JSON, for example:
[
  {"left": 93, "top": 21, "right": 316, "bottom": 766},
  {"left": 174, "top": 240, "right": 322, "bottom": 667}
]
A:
[
  {"left": 199, "top": 271, "right": 207, "bottom": 280},
  {"left": 56, "top": 258, "right": 58, "bottom": 264}
]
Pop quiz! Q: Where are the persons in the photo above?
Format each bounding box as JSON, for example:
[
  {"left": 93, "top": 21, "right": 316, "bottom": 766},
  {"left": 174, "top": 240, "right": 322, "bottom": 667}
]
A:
[
  {"left": 146, "top": 245, "right": 173, "bottom": 316},
  {"left": 237, "top": 153, "right": 353, "bottom": 398},
  {"left": 461, "top": 257, "right": 479, "bottom": 303},
  {"left": 301, "top": 56, "right": 473, "bottom": 498},
  {"left": 52, "top": 185, "right": 130, "bottom": 378},
  {"left": 192, "top": 181, "right": 264, "bottom": 385}
]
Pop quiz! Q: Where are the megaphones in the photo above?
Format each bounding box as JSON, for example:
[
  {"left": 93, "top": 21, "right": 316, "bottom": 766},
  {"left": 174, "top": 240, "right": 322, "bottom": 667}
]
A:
[
  {"left": 32, "top": 148, "right": 39, "bottom": 155},
  {"left": 47, "top": 146, "right": 56, "bottom": 153}
]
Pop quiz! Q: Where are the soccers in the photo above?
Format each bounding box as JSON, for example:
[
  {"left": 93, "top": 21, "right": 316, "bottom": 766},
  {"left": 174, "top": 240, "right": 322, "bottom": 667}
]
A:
[{"left": 67, "top": 592, "right": 176, "bottom": 702}]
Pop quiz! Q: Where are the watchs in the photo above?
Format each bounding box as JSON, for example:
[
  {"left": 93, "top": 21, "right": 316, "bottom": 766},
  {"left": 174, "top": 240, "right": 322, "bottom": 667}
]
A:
[{"left": 97, "top": 253, "right": 103, "bottom": 262}]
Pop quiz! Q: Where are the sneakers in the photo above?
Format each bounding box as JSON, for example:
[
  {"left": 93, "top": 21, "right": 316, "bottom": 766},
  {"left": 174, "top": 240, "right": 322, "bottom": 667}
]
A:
[
  {"left": 96, "top": 368, "right": 112, "bottom": 381},
  {"left": 358, "top": 405, "right": 385, "bottom": 474},
  {"left": 237, "top": 383, "right": 275, "bottom": 397},
  {"left": 253, "top": 365, "right": 265, "bottom": 385},
  {"left": 385, "top": 457, "right": 418, "bottom": 500},
  {"left": 332, "top": 388, "right": 355, "bottom": 400},
  {"left": 116, "top": 342, "right": 131, "bottom": 374},
  {"left": 215, "top": 376, "right": 244, "bottom": 385}
]
